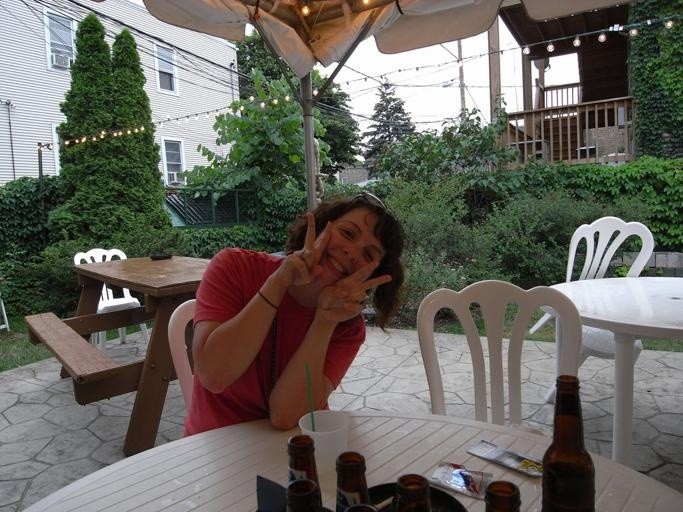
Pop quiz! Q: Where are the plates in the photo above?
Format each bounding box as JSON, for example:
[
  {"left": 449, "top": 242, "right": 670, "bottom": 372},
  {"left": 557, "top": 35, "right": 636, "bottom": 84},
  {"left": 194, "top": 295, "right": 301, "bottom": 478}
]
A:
[
  {"left": 365, "top": 481, "right": 468, "bottom": 512},
  {"left": 254, "top": 503, "right": 335, "bottom": 512}
]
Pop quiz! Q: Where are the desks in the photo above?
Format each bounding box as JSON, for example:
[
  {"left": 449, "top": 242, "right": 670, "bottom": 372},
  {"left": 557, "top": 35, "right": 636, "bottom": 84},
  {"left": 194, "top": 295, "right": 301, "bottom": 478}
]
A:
[
  {"left": 15, "top": 411, "right": 683, "bottom": 512},
  {"left": 24, "top": 255, "right": 211, "bottom": 457},
  {"left": 540, "top": 276, "right": 683, "bottom": 463}
]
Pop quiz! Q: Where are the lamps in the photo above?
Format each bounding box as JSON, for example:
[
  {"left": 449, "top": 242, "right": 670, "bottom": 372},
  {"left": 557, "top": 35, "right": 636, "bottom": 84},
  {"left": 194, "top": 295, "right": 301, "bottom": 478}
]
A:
[{"left": 520, "top": 15, "right": 675, "bottom": 57}]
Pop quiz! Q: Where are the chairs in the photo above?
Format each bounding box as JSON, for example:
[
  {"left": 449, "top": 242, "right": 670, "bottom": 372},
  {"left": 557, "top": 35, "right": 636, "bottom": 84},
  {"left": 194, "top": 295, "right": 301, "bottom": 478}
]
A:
[
  {"left": 71, "top": 247, "right": 155, "bottom": 349},
  {"left": 415, "top": 280, "right": 582, "bottom": 423},
  {"left": 168, "top": 297, "right": 204, "bottom": 418},
  {"left": 528, "top": 214, "right": 655, "bottom": 404}
]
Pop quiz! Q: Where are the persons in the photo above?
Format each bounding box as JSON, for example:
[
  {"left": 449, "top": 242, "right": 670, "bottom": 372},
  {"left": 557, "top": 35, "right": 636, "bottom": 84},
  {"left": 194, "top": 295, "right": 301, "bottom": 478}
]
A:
[{"left": 180, "top": 190, "right": 406, "bottom": 441}]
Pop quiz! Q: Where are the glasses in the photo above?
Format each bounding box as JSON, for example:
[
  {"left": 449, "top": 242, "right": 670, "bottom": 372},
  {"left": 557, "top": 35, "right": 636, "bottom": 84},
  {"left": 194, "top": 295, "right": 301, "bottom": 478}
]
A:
[{"left": 351, "top": 190, "right": 399, "bottom": 224}]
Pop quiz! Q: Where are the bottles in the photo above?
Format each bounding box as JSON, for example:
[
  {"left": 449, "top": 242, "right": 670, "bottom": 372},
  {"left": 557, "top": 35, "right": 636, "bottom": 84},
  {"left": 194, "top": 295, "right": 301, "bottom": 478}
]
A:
[
  {"left": 392, "top": 475, "right": 433, "bottom": 512},
  {"left": 284, "top": 479, "right": 325, "bottom": 512},
  {"left": 283, "top": 434, "right": 322, "bottom": 505},
  {"left": 484, "top": 479, "right": 522, "bottom": 512},
  {"left": 540, "top": 374, "right": 596, "bottom": 512},
  {"left": 335, "top": 449, "right": 378, "bottom": 512}
]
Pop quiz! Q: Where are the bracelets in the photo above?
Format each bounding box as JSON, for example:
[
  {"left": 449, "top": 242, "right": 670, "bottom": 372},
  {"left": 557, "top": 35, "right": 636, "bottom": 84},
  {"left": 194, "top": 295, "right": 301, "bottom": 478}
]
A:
[{"left": 255, "top": 289, "right": 280, "bottom": 312}]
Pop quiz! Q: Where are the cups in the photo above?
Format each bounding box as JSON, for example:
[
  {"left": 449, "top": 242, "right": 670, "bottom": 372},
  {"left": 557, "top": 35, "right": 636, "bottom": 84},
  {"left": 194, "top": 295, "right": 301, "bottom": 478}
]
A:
[{"left": 296, "top": 409, "right": 352, "bottom": 491}]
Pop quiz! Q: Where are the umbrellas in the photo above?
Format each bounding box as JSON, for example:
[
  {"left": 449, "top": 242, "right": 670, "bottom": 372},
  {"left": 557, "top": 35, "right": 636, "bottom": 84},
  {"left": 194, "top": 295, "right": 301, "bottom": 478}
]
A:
[{"left": 143, "top": 0, "right": 634, "bottom": 225}]
